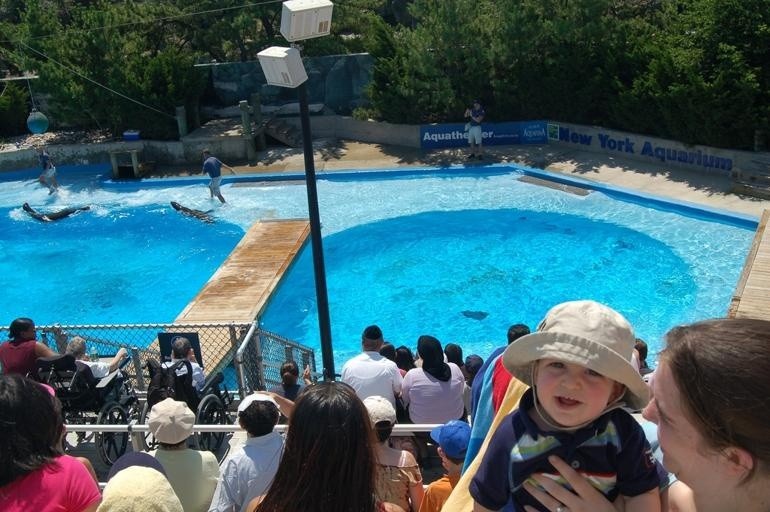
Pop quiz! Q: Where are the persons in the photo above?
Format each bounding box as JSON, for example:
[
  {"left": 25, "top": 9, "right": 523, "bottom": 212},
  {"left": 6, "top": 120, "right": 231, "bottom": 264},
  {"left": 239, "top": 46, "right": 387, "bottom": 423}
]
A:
[
  {"left": 466, "top": 298, "right": 770, "bottom": 512},
  {"left": 463, "top": 99, "right": 486, "bottom": 161},
  {"left": 37, "top": 146, "right": 59, "bottom": 195},
  {"left": 1, "top": 317, "right": 531, "bottom": 511},
  {"left": 201, "top": 149, "right": 237, "bottom": 204}
]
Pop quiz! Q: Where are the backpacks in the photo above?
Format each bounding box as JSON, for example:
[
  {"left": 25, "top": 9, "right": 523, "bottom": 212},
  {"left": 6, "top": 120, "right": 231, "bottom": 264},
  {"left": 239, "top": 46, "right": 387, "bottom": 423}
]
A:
[{"left": 146, "top": 357, "right": 192, "bottom": 405}]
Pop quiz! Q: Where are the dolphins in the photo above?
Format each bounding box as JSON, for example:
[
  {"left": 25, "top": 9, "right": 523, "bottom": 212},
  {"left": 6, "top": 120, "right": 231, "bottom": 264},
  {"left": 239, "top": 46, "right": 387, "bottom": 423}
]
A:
[
  {"left": 171, "top": 202, "right": 219, "bottom": 223},
  {"left": 23, "top": 203, "right": 90, "bottom": 221}
]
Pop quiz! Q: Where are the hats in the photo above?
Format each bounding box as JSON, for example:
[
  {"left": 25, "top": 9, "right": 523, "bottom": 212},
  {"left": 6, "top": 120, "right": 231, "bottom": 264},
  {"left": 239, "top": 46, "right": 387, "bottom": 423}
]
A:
[
  {"left": 431, "top": 419, "right": 470, "bottom": 459},
  {"left": 95, "top": 453, "right": 183, "bottom": 510},
  {"left": 501, "top": 300, "right": 650, "bottom": 410},
  {"left": 148, "top": 397, "right": 196, "bottom": 445},
  {"left": 466, "top": 355, "right": 484, "bottom": 374},
  {"left": 362, "top": 395, "right": 397, "bottom": 430}
]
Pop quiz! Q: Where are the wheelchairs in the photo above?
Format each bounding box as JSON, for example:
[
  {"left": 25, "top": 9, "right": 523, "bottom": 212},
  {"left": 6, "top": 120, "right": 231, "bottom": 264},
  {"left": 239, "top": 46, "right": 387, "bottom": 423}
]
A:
[
  {"left": 30, "top": 353, "right": 143, "bottom": 467},
  {"left": 139, "top": 357, "right": 234, "bottom": 452}
]
